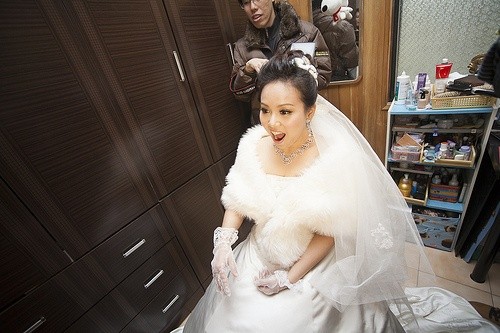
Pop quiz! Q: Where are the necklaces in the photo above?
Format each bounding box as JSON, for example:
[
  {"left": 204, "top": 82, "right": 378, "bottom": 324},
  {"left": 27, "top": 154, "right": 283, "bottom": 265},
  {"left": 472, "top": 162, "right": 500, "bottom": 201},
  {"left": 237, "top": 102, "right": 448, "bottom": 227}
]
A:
[{"left": 272, "top": 126, "right": 313, "bottom": 164}]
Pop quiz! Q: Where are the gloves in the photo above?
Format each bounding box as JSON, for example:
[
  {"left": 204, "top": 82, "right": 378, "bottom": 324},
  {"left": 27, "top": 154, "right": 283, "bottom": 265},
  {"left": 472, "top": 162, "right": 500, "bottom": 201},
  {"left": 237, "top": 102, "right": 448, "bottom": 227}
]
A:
[
  {"left": 254, "top": 267, "right": 292, "bottom": 295},
  {"left": 211, "top": 225, "right": 239, "bottom": 295}
]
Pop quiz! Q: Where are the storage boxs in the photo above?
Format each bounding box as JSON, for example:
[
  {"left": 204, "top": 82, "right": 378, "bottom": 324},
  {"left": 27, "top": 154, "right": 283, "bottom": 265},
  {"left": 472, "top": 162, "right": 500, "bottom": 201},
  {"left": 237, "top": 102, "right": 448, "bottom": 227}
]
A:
[
  {"left": 390, "top": 142, "right": 421, "bottom": 161},
  {"left": 429, "top": 184, "right": 460, "bottom": 203},
  {"left": 406, "top": 213, "right": 461, "bottom": 252}
]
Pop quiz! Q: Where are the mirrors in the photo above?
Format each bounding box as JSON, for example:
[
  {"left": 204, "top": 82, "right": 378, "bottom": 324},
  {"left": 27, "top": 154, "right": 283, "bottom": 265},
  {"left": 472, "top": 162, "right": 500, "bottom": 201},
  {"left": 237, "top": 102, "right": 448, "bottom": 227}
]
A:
[{"left": 309, "top": 0, "right": 365, "bottom": 86}]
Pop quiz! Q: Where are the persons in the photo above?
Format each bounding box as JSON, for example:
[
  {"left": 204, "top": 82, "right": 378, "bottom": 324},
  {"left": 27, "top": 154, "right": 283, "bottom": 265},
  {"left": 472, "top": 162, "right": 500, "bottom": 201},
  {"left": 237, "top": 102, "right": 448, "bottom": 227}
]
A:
[
  {"left": 183, "top": 65, "right": 409, "bottom": 333},
  {"left": 312, "top": 0, "right": 359, "bottom": 81},
  {"left": 229, "top": 0, "right": 333, "bottom": 125}
]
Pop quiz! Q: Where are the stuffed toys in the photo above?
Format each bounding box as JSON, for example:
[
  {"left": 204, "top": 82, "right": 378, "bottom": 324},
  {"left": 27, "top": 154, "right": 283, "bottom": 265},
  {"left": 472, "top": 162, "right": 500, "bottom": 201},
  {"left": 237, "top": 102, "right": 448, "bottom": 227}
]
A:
[{"left": 321, "top": 0, "right": 353, "bottom": 21}]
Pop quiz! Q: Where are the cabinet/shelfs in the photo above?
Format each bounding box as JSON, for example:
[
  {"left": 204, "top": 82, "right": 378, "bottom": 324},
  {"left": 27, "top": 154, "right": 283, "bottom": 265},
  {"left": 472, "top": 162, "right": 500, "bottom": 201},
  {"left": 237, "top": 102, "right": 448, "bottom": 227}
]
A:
[
  {"left": 0, "top": 0, "right": 253, "bottom": 333},
  {"left": 385, "top": 96, "right": 500, "bottom": 252}
]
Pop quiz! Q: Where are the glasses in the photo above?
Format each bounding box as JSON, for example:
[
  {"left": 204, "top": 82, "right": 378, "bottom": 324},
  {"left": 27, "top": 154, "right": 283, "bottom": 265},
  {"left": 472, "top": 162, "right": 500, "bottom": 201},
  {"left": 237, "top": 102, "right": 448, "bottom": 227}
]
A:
[{"left": 239, "top": 0, "right": 250, "bottom": 10}]
